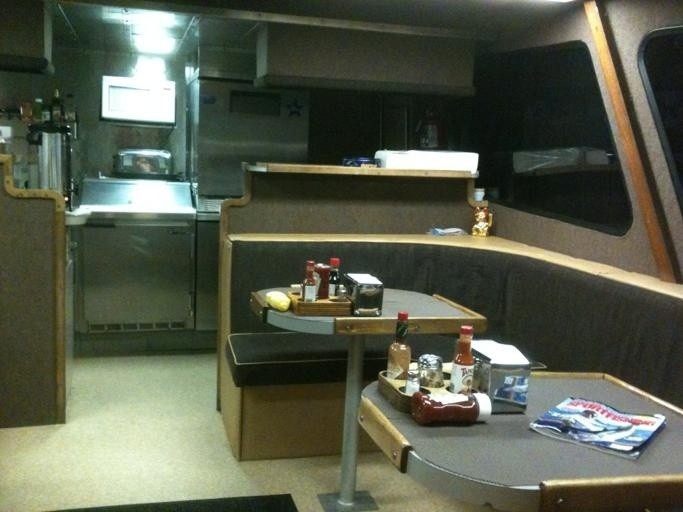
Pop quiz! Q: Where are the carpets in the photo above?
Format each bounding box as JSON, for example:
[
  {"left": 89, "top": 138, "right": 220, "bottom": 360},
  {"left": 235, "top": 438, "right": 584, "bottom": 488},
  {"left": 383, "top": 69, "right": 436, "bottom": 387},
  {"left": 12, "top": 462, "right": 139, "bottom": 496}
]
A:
[{"left": 47, "top": 493, "right": 298, "bottom": 511}]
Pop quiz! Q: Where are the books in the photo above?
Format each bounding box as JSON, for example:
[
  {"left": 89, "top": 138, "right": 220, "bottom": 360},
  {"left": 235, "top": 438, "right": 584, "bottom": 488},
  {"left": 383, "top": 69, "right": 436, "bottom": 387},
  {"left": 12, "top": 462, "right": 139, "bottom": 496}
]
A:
[{"left": 528, "top": 396, "right": 666, "bottom": 462}]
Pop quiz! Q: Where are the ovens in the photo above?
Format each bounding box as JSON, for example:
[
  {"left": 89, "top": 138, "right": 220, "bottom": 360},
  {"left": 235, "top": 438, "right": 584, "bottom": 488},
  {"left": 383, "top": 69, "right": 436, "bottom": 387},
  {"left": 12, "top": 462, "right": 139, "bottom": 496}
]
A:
[{"left": 81, "top": 219, "right": 196, "bottom": 333}]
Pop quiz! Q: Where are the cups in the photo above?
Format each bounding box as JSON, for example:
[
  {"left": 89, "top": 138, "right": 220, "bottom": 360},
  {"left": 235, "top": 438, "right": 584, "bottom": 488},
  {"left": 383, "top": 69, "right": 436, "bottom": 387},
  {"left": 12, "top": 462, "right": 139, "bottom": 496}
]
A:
[
  {"left": 417, "top": 354, "right": 444, "bottom": 387},
  {"left": 291, "top": 284, "right": 301, "bottom": 295},
  {"left": 474, "top": 188, "right": 486, "bottom": 202}
]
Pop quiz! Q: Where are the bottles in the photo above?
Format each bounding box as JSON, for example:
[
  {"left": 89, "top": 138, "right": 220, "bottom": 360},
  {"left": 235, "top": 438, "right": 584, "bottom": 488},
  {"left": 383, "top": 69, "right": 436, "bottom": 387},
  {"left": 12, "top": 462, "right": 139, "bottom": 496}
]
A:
[
  {"left": 337, "top": 284, "right": 348, "bottom": 302},
  {"left": 405, "top": 369, "right": 420, "bottom": 394},
  {"left": 449, "top": 325, "right": 474, "bottom": 394},
  {"left": 64, "top": 94, "right": 76, "bottom": 122},
  {"left": 52, "top": 89, "right": 61, "bottom": 122},
  {"left": 303, "top": 261, "right": 317, "bottom": 303},
  {"left": 266, "top": 291, "right": 290, "bottom": 311},
  {"left": 328, "top": 258, "right": 341, "bottom": 298},
  {"left": 387, "top": 312, "right": 411, "bottom": 380},
  {"left": 412, "top": 392, "right": 492, "bottom": 426},
  {"left": 33, "top": 98, "right": 43, "bottom": 121}
]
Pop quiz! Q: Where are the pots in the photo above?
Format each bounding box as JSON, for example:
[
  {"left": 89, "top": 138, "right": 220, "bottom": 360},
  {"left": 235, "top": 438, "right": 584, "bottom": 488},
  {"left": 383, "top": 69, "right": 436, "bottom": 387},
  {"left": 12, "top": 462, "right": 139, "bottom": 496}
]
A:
[{"left": 111, "top": 149, "right": 184, "bottom": 180}]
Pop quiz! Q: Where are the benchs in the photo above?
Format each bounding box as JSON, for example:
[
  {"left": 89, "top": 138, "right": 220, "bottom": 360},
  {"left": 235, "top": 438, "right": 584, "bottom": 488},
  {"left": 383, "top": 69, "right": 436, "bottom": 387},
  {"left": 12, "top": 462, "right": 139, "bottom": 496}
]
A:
[{"left": 218, "top": 230, "right": 683, "bottom": 462}]
